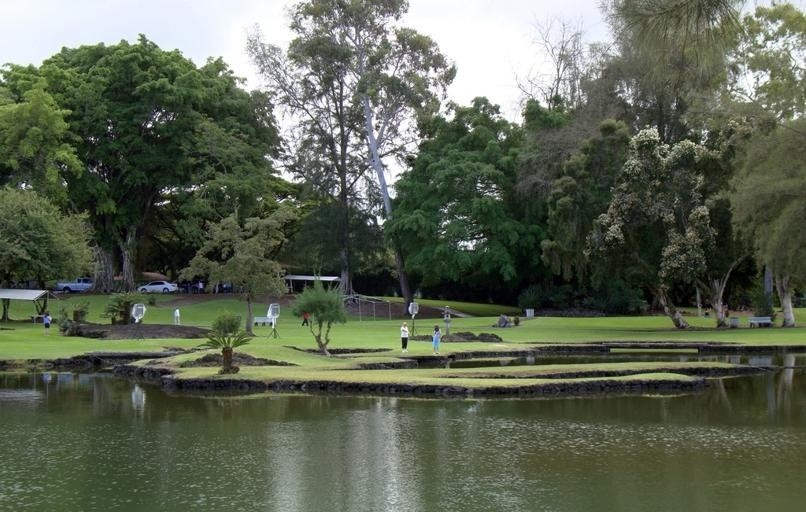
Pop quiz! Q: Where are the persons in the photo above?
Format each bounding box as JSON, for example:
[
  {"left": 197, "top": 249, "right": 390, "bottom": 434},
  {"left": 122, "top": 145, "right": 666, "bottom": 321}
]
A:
[
  {"left": 43, "top": 311, "right": 52, "bottom": 334},
  {"left": 302, "top": 310, "right": 310, "bottom": 326},
  {"left": 432, "top": 324, "right": 442, "bottom": 355},
  {"left": 401, "top": 322, "right": 409, "bottom": 352},
  {"left": 174, "top": 306, "right": 181, "bottom": 325}
]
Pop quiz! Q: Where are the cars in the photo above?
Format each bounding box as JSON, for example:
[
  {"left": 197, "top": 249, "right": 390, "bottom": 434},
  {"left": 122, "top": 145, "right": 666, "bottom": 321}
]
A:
[{"left": 137, "top": 280, "right": 180, "bottom": 296}]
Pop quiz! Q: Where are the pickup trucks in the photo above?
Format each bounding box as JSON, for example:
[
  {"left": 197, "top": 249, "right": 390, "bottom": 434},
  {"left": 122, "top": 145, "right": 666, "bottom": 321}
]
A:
[{"left": 55, "top": 277, "right": 95, "bottom": 295}]
